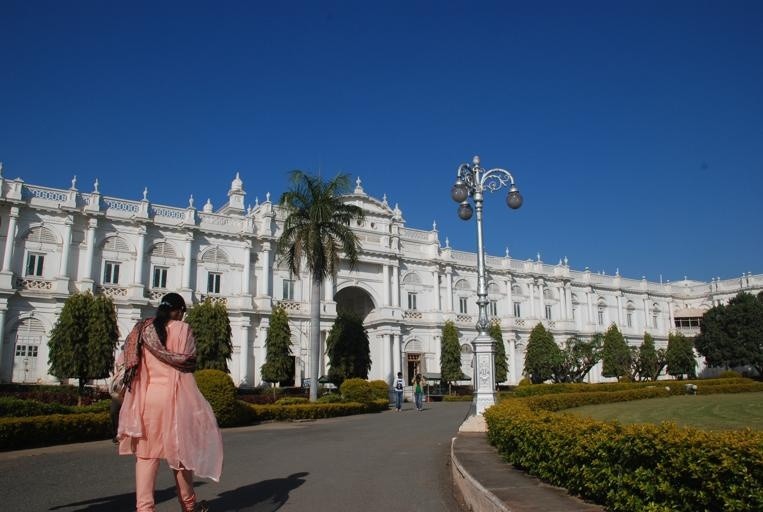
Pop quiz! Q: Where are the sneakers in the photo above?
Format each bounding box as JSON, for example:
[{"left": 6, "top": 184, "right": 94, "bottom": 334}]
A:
[
  {"left": 394, "top": 408, "right": 403, "bottom": 412},
  {"left": 415, "top": 406, "right": 423, "bottom": 411}
]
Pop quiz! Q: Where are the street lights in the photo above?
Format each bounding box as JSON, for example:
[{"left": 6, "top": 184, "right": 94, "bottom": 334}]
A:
[{"left": 452, "top": 155, "right": 523, "bottom": 418}]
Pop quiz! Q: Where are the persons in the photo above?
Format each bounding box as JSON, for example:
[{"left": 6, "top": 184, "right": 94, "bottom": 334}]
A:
[
  {"left": 410, "top": 373, "right": 425, "bottom": 411},
  {"left": 109, "top": 293, "right": 223, "bottom": 511},
  {"left": 392, "top": 372, "right": 406, "bottom": 411}
]
